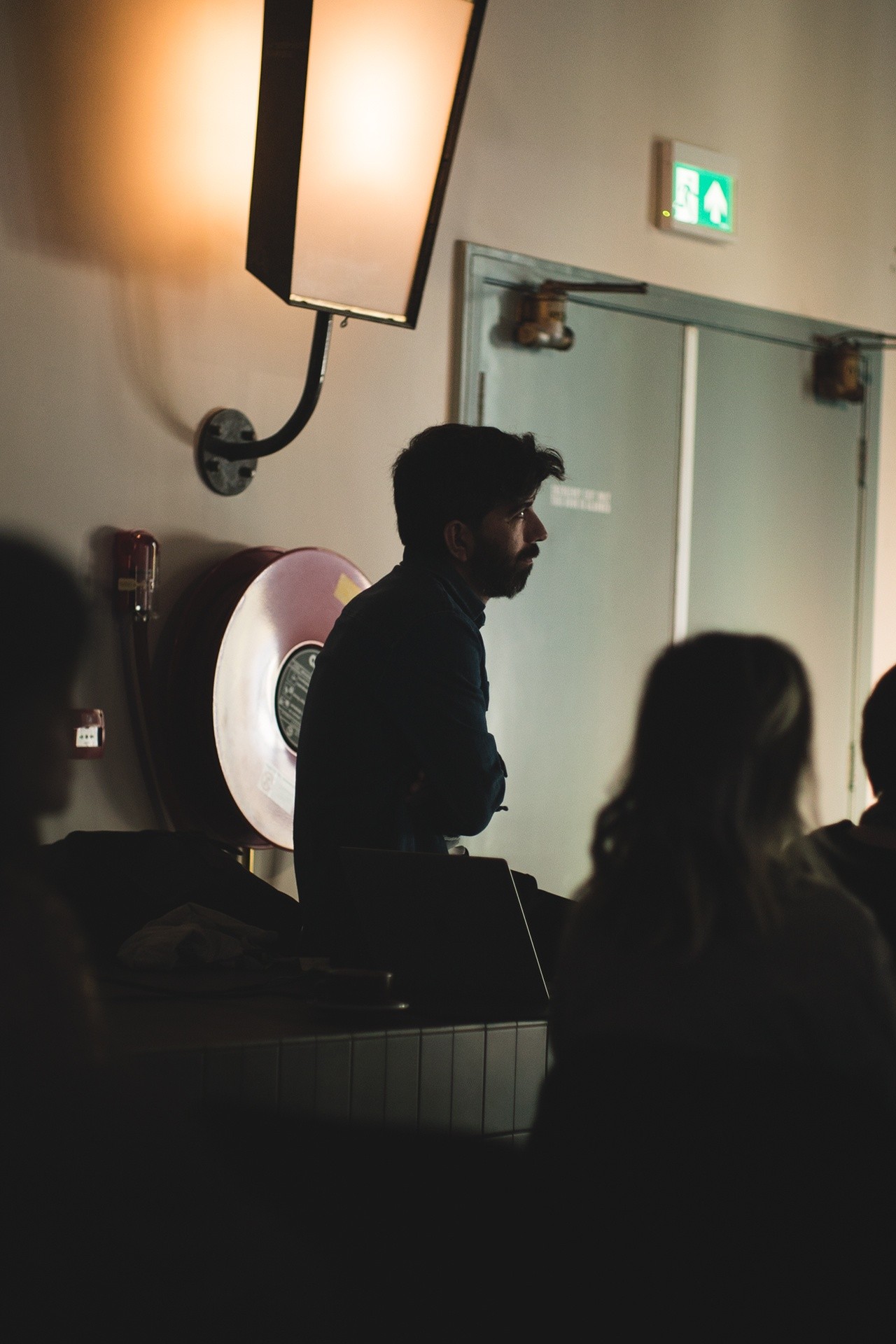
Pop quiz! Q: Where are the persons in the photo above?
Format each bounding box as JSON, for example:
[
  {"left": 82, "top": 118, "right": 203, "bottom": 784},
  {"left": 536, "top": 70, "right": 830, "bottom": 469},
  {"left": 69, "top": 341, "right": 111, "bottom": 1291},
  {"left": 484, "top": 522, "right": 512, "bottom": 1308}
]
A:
[
  {"left": 804, "top": 665, "right": 896, "bottom": 946},
  {"left": 527, "top": 630, "right": 896, "bottom": 1344},
  {"left": 293, "top": 422, "right": 575, "bottom": 1017}
]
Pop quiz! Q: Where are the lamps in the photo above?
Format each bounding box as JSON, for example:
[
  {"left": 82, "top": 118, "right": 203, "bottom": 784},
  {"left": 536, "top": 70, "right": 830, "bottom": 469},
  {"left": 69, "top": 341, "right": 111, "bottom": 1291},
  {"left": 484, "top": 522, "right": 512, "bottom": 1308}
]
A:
[{"left": 196, "top": 1, "right": 487, "bottom": 496}]
